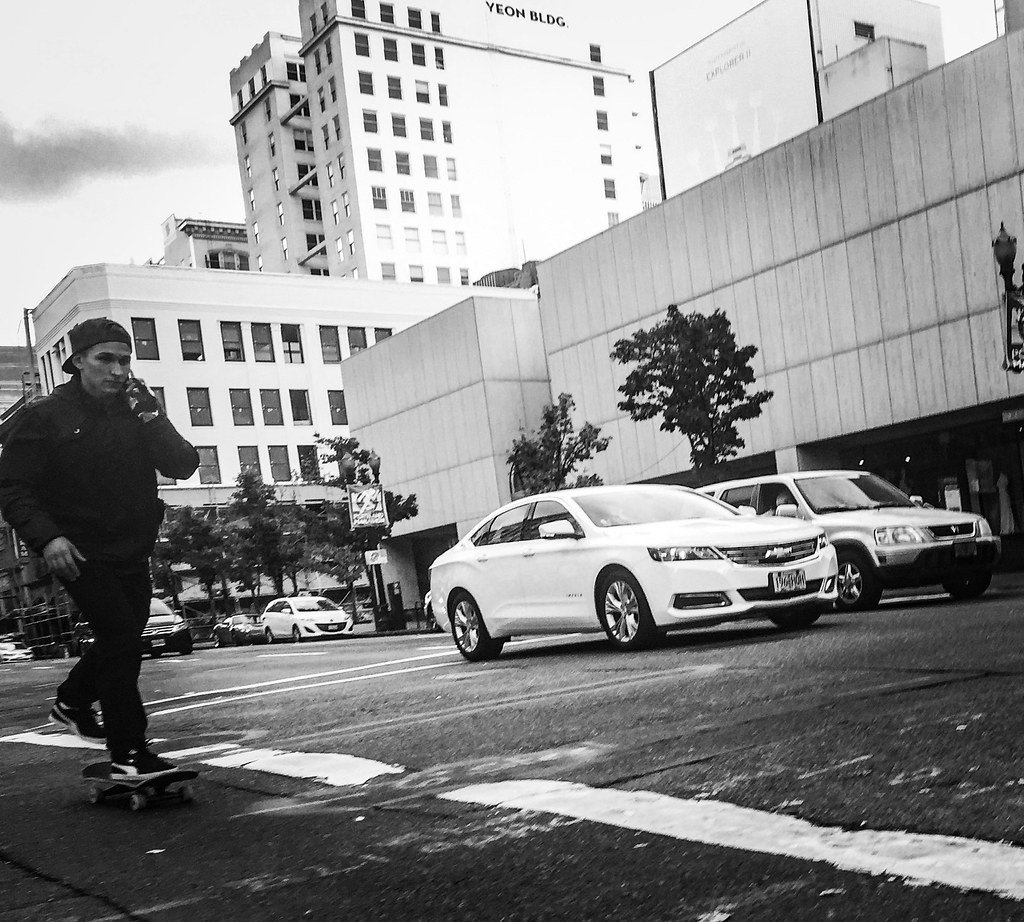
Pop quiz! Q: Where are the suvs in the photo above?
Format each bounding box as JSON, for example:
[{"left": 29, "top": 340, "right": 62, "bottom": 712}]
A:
[{"left": 695, "top": 470, "right": 1003, "bottom": 613}]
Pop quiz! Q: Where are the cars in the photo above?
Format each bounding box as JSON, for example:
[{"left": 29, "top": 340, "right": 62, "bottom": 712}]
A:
[
  {"left": 1, "top": 641, "right": 34, "bottom": 663},
  {"left": 259, "top": 597, "right": 355, "bottom": 643},
  {"left": 211, "top": 613, "right": 268, "bottom": 646},
  {"left": 427, "top": 485, "right": 839, "bottom": 662},
  {"left": 73, "top": 598, "right": 193, "bottom": 660}
]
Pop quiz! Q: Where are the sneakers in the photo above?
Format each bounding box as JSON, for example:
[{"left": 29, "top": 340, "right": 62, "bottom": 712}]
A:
[
  {"left": 108, "top": 736, "right": 179, "bottom": 780},
  {"left": 48, "top": 695, "right": 108, "bottom": 744}
]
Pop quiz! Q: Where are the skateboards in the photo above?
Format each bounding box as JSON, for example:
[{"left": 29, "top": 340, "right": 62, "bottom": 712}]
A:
[{"left": 80, "top": 759, "right": 201, "bottom": 813}]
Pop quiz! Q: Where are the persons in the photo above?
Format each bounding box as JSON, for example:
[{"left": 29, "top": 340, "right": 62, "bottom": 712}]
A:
[{"left": 0, "top": 317, "right": 202, "bottom": 780}]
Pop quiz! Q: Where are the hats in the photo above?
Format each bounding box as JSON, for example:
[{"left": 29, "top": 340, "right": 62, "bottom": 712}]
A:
[{"left": 61, "top": 317, "right": 131, "bottom": 375}]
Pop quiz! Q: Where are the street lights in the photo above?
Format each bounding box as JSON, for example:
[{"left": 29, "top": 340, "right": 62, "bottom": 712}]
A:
[{"left": 992, "top": 222, "right": 1024, "bottom": 349}]
